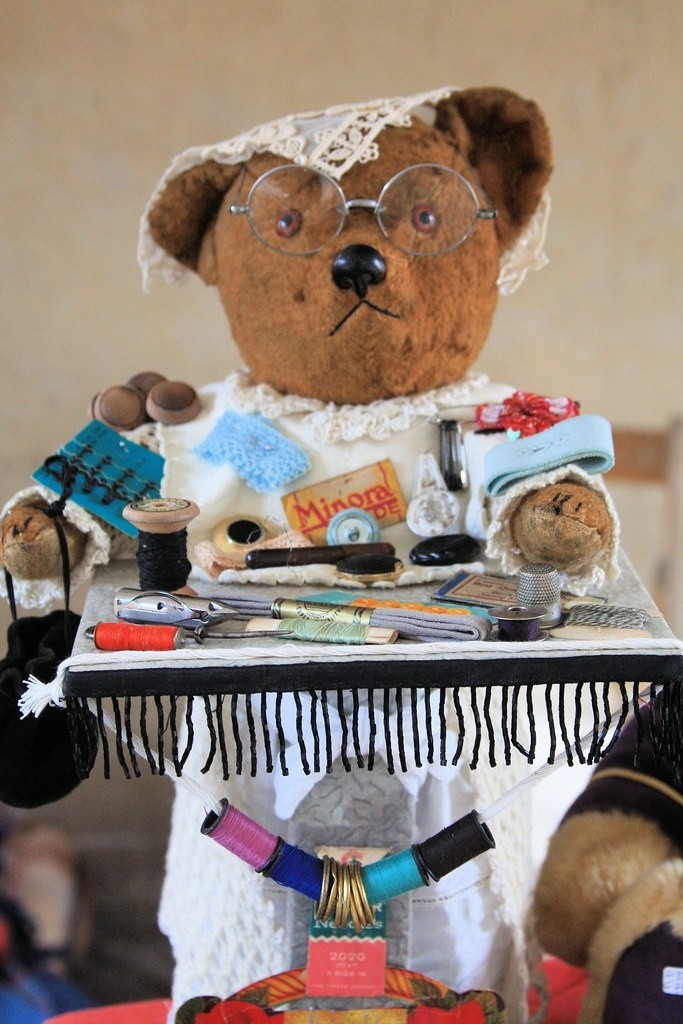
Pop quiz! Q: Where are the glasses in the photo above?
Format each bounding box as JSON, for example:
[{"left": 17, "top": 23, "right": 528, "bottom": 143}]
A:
[{"left": 230, "top": 166, "right": 499, "bottom": 257}]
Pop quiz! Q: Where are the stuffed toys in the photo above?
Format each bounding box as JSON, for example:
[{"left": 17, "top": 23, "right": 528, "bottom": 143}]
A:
[
  {"left": 531, "top": 675, "right": 683, "bottom": 1024},
  {"left": 0, "top": 84, "right": 661, "bottom": 1024}
]
